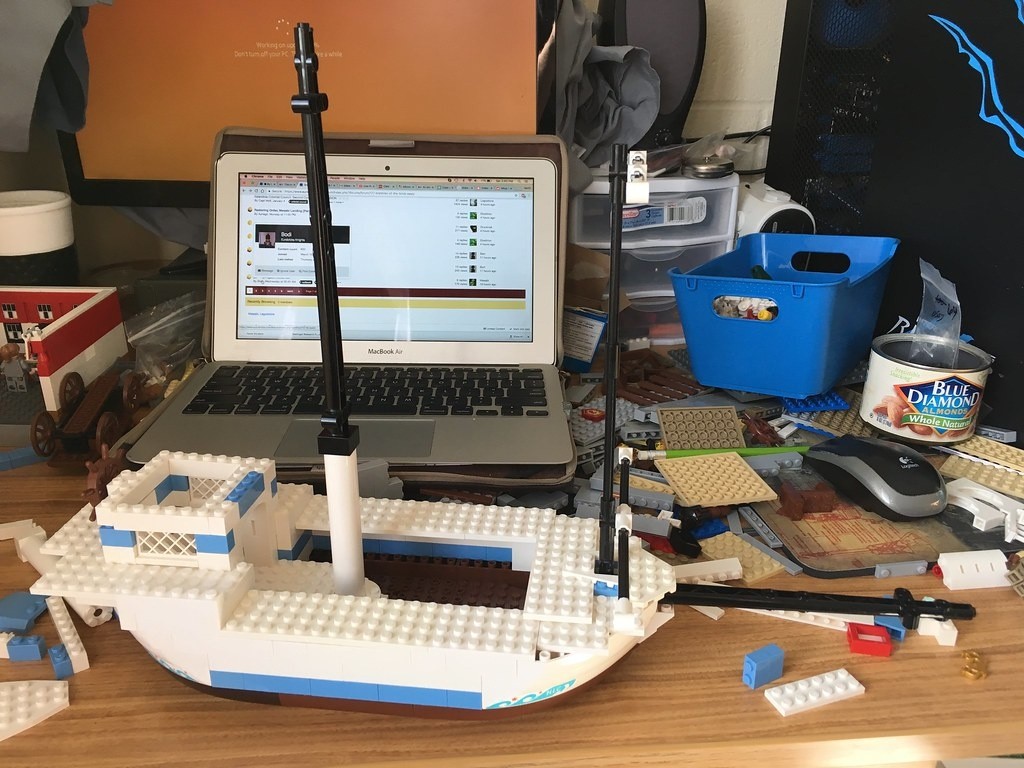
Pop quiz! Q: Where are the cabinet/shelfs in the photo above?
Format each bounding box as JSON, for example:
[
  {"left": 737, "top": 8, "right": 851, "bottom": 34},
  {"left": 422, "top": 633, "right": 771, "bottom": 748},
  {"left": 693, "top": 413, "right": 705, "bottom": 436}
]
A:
[{"left": 568, "top": 174, "right": 740, "bottom": 344}]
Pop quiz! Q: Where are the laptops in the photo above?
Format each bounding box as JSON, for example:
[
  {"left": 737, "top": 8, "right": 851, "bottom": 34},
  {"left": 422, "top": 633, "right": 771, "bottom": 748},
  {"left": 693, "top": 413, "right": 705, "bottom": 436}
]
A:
[{"left": 125, "top": 151, "right": 574, "bottom": 465}]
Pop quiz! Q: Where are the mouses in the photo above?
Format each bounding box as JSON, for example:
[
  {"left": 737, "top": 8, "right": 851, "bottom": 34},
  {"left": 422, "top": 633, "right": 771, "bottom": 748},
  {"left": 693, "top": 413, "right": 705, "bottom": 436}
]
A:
[{"left": 804, "top": 433, "right": 948, "bottom": 521}]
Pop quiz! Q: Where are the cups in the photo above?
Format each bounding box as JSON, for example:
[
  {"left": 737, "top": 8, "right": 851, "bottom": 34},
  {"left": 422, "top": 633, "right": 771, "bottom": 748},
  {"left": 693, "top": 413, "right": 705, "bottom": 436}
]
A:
[{"left": 0, "top": 190, "right": 79, "bottom": 286}]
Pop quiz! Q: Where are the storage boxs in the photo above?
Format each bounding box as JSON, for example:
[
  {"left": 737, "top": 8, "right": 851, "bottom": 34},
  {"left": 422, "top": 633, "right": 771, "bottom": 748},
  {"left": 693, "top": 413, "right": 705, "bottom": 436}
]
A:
[{"left": 669, "top": 234, "right": 902, "bottom": 400}]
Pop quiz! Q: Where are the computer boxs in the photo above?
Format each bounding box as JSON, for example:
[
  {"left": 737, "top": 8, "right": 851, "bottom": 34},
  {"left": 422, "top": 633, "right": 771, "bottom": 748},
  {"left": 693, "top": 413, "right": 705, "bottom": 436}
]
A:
[{"left": 763, "top": 0, "right": 1024, "bottom": 448}]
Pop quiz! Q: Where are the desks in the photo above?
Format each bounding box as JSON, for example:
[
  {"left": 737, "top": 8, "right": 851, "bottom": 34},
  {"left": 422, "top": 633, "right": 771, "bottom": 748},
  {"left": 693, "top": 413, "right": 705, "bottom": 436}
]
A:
[{"left": 0, "top": 438, "right": 1024, "bottom": 768}]
